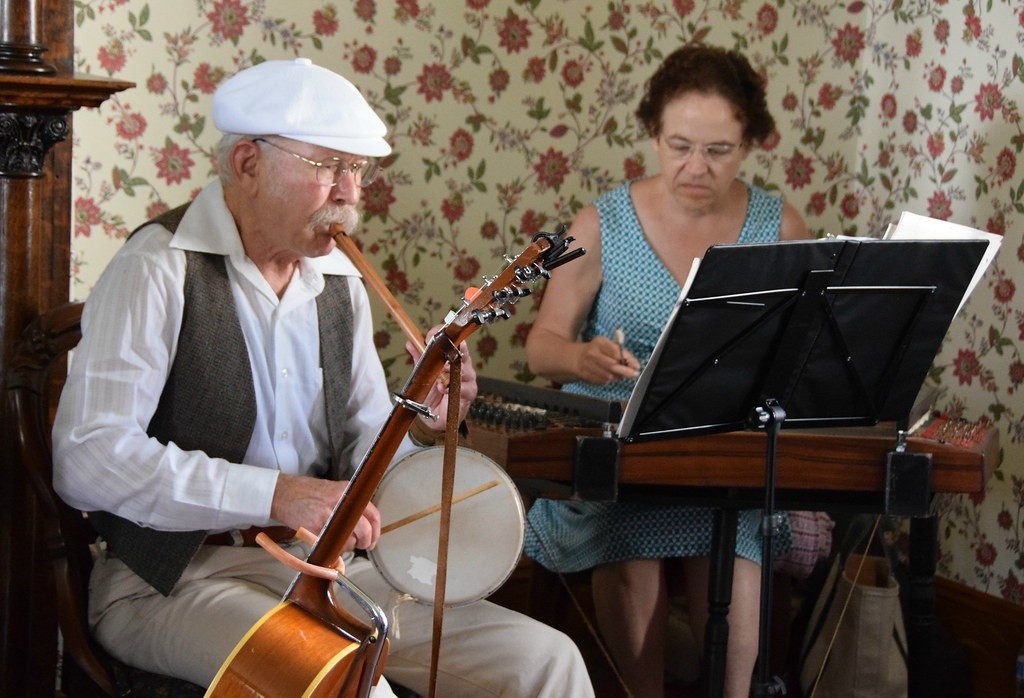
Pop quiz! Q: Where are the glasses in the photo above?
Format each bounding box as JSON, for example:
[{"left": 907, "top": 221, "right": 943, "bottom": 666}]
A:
[
  {"left": 251, "top": 139, "right": 384, "bottom": 188},
  {"left": 656, "top": 128, "right": 749, "bottom": 164}
]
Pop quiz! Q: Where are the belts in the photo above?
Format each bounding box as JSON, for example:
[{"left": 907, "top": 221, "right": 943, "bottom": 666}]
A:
[{"left": 204, "top": 525, "right": 296, "bottom": 549}]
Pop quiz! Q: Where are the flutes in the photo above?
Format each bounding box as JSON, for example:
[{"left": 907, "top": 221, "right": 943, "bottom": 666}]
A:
[{"left": 329, "top": 222, "right": 453, "bottom": 388}]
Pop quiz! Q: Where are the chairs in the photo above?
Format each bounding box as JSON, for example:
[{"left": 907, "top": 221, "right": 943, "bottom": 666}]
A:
[{"left": 3, "top": 304, "right": 208, "bottom": 698}]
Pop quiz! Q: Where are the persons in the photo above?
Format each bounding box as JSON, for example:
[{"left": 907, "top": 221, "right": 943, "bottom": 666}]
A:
[
  {"left": 51, "top": 59, "right": 595, "bottom": 698},
  {"left": 522, "top": 45, "right": 809, "bottom": 698}
]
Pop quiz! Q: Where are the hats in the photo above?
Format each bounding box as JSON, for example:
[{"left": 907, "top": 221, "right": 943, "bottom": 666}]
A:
[{"left": 213, "top": 58, "right": 393, "bottom": 157}]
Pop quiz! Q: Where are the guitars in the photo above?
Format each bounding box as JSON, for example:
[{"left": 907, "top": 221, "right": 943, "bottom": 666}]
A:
[{"left": 200, "top": 224, "right": 588, "bottom": 697}]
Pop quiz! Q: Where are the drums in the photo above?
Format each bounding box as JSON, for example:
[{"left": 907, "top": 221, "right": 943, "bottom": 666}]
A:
[{"left": 365, "top": 443, "right": 529, "bottom": 605}]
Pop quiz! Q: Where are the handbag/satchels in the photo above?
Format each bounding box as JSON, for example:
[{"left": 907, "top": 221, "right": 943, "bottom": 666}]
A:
[{"left": 798, "top": 514, "right": 912, "bottom": 698}]
